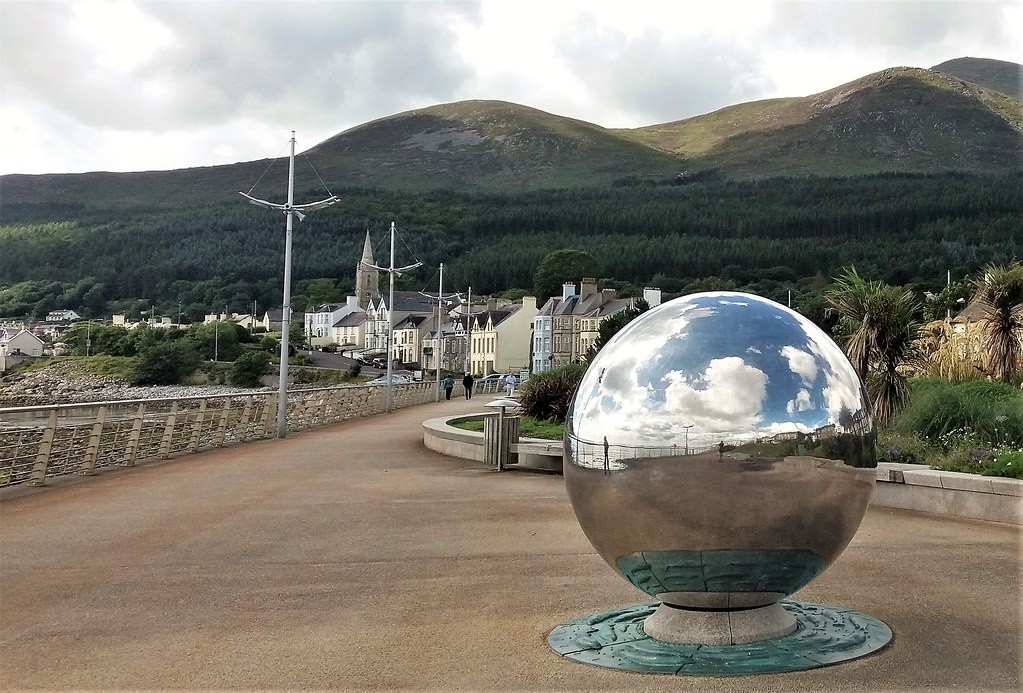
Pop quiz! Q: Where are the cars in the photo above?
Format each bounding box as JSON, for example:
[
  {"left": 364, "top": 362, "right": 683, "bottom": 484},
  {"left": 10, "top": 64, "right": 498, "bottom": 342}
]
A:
[
  {"left": 362, "top": 373, "right": 413, "bottom": 385},
  {"left": 295, "top": 342, "right": 313, "bottom": 350},
  {"left": 372, "top": 358, "right": 388, "bottom": 369},
  {"left": 358, "top": 356, "right": 371, "bottom": 366}
]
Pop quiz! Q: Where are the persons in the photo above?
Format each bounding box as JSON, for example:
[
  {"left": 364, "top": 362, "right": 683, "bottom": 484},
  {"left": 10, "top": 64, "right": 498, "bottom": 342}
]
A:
[
  {"left": 443, "top": 375, "right": 453, "bottom": 400},
  {"left": 506, "top": 372, "right": 516, "bottom": 396},
  {"left": 463, "top": 372, "right": 473, "bottom": 400},
  {"left": 603, "top": 436, "right": 610, "bottom": 476},
  {"left": 719, "top": 441, "right": 725, "bottom": 457}
]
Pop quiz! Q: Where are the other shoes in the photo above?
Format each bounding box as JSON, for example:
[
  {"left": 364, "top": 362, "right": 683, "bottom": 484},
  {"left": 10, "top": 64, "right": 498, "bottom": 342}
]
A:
[
  {"left": 466, "top": 399, "right": 468, "bottom": 400},
  {"left": 446, "top": 399, "right": 450, "bottom": 400},
  {"left": 469, "top": 396, "right": 471, "bottom": 399}
]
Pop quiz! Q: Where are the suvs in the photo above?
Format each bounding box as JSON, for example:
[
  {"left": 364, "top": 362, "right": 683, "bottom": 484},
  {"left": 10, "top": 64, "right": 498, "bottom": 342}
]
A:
[
  {"left": 474, "top": 373, "right": 500, "bottom": 387},
  {"left": 498, "top": 374, "right": 520, "bottom": 389}
]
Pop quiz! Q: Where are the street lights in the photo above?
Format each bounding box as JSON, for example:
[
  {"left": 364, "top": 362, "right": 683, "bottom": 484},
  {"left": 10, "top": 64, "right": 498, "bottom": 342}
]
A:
[
  {"left": 359, "top": 259, "right": 422, "bottom": 413},
  {"left": 237, "top": 190, "right": 339, "bottom": 438},
  {"left": 418, "top": 291, "right": 464, "bottom": 400},
  {"left": 682, "top": 424, "right": 694, "bottom": 458},
  {"left": 452, "top": 309, "right": 486, "bottom": 376}
]
